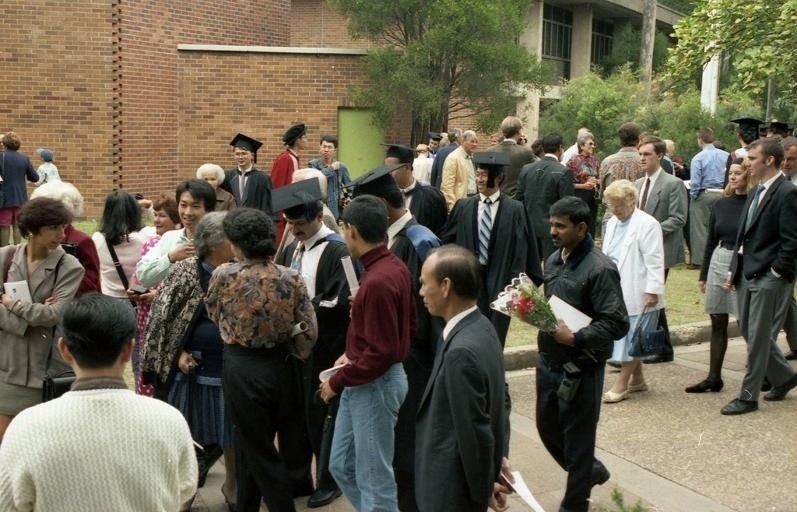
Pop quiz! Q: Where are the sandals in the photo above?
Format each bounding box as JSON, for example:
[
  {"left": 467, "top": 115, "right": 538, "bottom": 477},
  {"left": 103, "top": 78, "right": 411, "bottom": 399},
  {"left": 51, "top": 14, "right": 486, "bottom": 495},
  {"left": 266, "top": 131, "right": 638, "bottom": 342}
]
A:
[
  {"left": 601, "top": 388, "right": 628, "bottom": 402},
  {"left": 627, "top": 380, "right": 647, "bottom": 392}
]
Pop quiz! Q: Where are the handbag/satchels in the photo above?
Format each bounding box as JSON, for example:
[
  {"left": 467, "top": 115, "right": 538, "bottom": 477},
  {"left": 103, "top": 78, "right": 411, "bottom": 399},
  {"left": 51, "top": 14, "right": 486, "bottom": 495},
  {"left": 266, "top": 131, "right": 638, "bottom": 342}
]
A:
[
  {"left": 594, "top": 184, "right": 603, "bottom": 205},
  {"left": 42, "top": 375, "right": 76, "bottom": 403},
  {"left": 628, "top": 327, "right": 665, "bottom": 356}
]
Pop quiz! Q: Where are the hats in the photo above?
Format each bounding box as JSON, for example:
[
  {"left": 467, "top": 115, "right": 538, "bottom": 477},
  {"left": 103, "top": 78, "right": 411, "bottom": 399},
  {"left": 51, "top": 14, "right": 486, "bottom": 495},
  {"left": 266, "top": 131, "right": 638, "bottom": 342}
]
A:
[
  {"left": 283, "top": 124, "right": 307, "bottom": 145},
  {"left": 271, "top": 177, "right": 322, "bottom": 219},
  {"left": 428, "top": 131, "right": 441, "bottom": 141},
  {"left": 379, "top": 143, "right": 424, "bottom": 162},
  {"left": 730, "top": 118, "right": 794, "bottom": 139},
  {"left": 230, "top": 133, "right": 263, "bottom": 163},
  {"left": 36, "top": 149, "right": 53, "bottom": 160},
  {"left": 474, "top": 152, "right": 511, "bottom": 188},
  {"left": 340, "top": 163, "right": 406, "bottom": 197}
]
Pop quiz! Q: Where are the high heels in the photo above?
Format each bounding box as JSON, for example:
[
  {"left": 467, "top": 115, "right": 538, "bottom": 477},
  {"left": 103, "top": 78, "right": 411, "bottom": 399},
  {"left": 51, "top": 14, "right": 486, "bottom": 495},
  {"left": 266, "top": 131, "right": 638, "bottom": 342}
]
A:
[
  {"left": 221, "top": 483, "right": 238, "bottom": 512},
  {"left": 686, "top": 375, "right": 723, "bottom": 392}
]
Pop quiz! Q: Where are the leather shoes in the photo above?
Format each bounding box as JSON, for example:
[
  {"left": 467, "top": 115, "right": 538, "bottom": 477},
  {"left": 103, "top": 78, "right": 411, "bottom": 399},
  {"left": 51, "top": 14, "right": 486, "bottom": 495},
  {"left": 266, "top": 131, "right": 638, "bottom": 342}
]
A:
[
  {"left": 607, "top": 362, "right": 622, "bottom": 368},
  {"left": 308, "top": 489, "right": 341, "bottom": 507},
  {"left": 764, "top": 373, "right": 797, "bottom": 400},
  {"left": 686, "top": 264, "right": 704, "bottom": 269},
  {"left": 784, "top": 349, "right": 797, "bottom": 360},
  {"left": 558, "top": 458, "right": 610, "bottom": 512},
  {"left": 644, "top": 353, "right": 673, "bottom": 363},
  {"left": 722, "top": 398, "right": 757, "bottom": 414}
]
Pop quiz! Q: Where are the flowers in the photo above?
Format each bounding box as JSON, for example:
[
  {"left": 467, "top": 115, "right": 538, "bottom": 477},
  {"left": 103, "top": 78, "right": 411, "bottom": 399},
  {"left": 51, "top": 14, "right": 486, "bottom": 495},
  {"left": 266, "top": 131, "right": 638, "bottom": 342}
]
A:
[{"left": 486, "top": 271, "right": 600, "bottom": 365}]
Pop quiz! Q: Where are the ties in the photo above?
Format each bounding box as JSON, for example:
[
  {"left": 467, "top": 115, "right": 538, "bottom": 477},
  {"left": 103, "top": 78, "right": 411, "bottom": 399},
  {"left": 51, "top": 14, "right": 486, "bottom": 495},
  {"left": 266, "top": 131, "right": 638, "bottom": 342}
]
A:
[
  {"left": 479, "top": 199, "right": 492, "bottom": 265},
  {"left": 240, "top": 168, "right": 246, "bottom": 200},
  {"left": 744, "top": 184, "right": 764, "bottom": 236},
  {"left": 292, "top": 242, "right": 304, "bottom": 270},
  {"left": 640, "top": 178, "right": 650, "bottom": 210}
]
